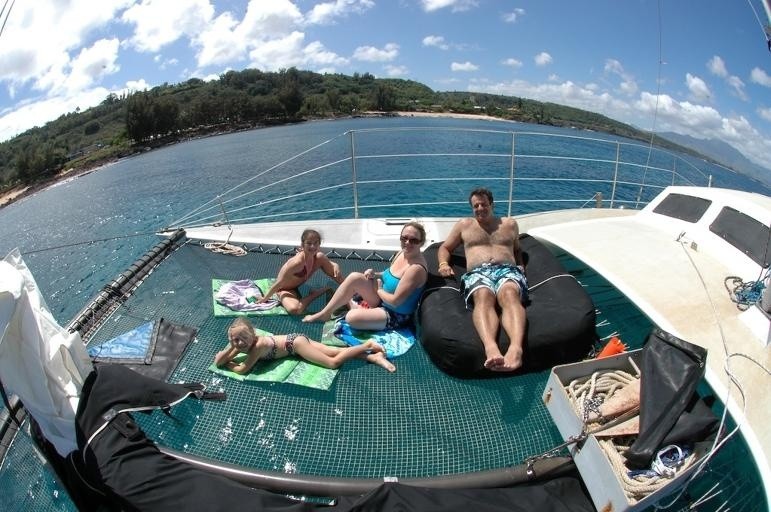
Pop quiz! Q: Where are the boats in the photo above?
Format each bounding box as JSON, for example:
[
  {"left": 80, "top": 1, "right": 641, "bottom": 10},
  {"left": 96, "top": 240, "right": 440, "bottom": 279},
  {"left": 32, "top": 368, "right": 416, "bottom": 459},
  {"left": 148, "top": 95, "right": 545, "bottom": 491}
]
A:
[{"left": 0, "top": 2, "right": 771, "bottom": 508}]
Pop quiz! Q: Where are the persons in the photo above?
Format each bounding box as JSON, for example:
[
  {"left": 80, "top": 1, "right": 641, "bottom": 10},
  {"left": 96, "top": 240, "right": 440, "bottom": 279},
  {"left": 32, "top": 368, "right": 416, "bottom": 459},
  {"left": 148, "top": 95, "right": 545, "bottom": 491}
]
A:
[
  {"left": 302, "top": 221, "right": 430, "bottom": 334},
  {"left": 437, "top": 188, "right": 531, "bottom": 373},
  {"left": 214, "top": 319, "right": 396, "bottom": 376},
  {"left": 254, "top": 228, "right": 350, "bottom": 316}
]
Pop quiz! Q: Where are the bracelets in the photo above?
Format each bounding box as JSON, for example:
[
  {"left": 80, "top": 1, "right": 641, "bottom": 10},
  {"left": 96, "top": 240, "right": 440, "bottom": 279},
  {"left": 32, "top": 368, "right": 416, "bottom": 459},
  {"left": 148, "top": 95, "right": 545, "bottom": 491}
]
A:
[{"left": 439, "top": 261, "right": 448, "bottom": 267}]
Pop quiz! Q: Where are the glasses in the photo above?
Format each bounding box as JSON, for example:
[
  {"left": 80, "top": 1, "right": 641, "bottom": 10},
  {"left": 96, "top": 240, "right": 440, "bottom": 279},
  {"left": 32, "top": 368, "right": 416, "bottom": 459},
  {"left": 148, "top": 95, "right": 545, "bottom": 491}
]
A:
[{"left": 400, "top": 237, "right": 422, "bottom": 244}]
